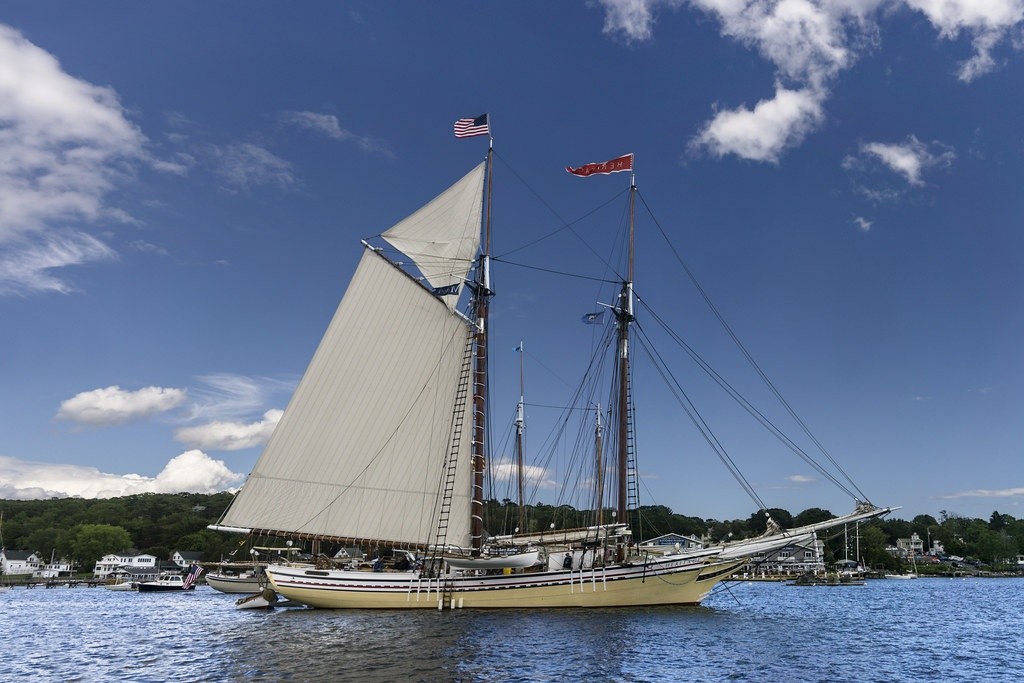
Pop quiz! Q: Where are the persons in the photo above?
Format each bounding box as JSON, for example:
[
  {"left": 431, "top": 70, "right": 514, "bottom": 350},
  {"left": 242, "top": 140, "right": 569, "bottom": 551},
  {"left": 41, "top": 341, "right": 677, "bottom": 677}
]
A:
[
  {"left": 394, "top": 554, "right": 411, "bottom": 570},
  {"left": 615, "top": 545, "right": 624, "bottom": 563},
  {"left": 592, "top": 557, "right": 603, "bottom": 568},
  {"left": 413, "top": 561, "right": 422, "bottom": 578},
  {"left": 373, "top": 558, "right": 385, "bottom": 572},
  {"left": 563, "top": 552, "right": 572, "bottom": 568},
  {"left": 604, "top": 548, "right": 609, "bottom": 563}
]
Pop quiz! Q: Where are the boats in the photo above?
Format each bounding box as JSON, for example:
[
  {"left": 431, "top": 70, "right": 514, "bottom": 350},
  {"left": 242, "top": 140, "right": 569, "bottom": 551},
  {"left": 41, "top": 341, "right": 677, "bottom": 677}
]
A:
[
  {"left": 105, "top": 581, "right": 140, "bottom": 591},
  {"left": 205, "top": 528, "right": 319, "bottom": 594},
  {"left": 137, "top": 575, "right": 196, "bottom": 591},
  {"left": 785, "top": 568, "right": 866, "bottom": 586}
]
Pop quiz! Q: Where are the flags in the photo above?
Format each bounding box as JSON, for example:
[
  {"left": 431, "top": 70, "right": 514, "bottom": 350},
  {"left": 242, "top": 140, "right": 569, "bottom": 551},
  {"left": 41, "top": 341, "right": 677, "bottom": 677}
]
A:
[
  {"left": 182, "top": 564, "right": 204, "bottom": 590},
  {"left": 512, "top": 345, "right": 521, "bottom": 352},
  {"left": 582, "top": 311, "right": 604, "bottom": 325},
  {"left": 453, "top": 113, "right": 489, "bottom": 138}
]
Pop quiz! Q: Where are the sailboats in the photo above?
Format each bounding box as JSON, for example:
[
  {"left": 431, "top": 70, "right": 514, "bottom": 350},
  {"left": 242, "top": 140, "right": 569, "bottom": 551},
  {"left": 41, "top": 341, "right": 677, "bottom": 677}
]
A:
[{"left": 205, "top": 144, "right": 903, "bottom": 613}]
